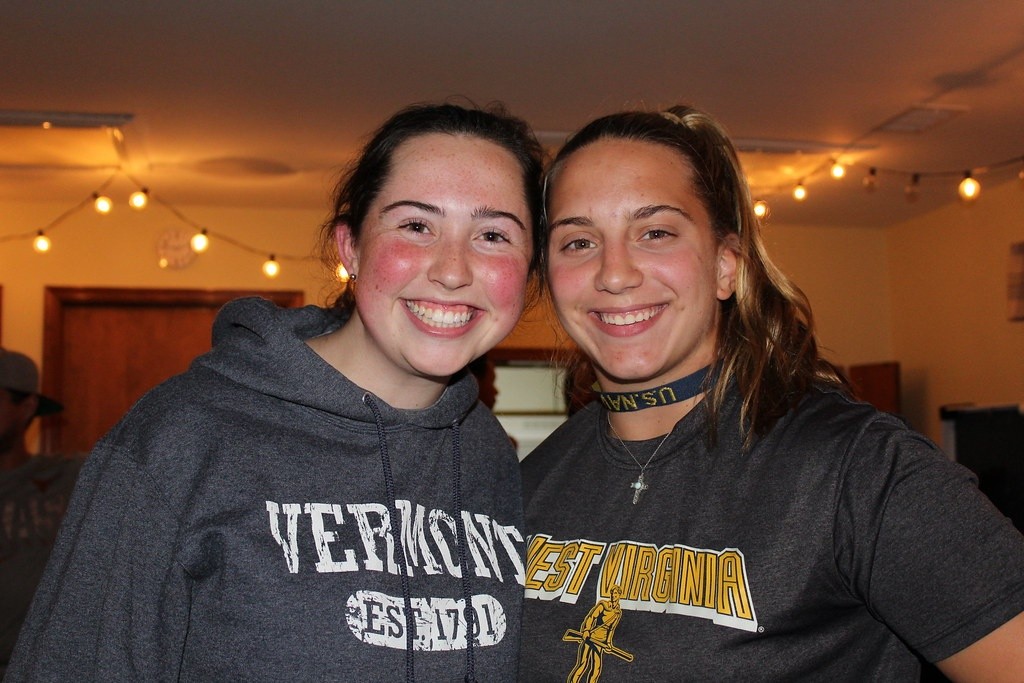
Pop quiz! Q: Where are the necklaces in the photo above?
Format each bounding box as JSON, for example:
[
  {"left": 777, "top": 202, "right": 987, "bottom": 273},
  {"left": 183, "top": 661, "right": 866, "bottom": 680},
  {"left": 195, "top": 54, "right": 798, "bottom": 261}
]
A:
[{"left": 607, "top": 412, "right": 672, "bottom": 504}]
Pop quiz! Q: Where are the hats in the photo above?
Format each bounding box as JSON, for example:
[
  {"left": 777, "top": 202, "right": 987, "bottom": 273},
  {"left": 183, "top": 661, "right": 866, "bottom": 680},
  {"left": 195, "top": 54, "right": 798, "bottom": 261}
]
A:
[{"left": 0, "top": 350, "right": 64, "bottom": 413}]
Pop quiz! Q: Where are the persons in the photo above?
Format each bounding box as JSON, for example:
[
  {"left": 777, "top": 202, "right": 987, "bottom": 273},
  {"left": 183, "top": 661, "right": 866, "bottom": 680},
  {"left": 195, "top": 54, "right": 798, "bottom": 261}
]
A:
[
  {"left": 517, "top": 106, "right": 1024, "bottom": 683},
  {"left": 0, "top": 94, "right": 554, "bottom": 683},
  {"left": 0, "top": 351, "right": 82, "bottom": 660}
]
